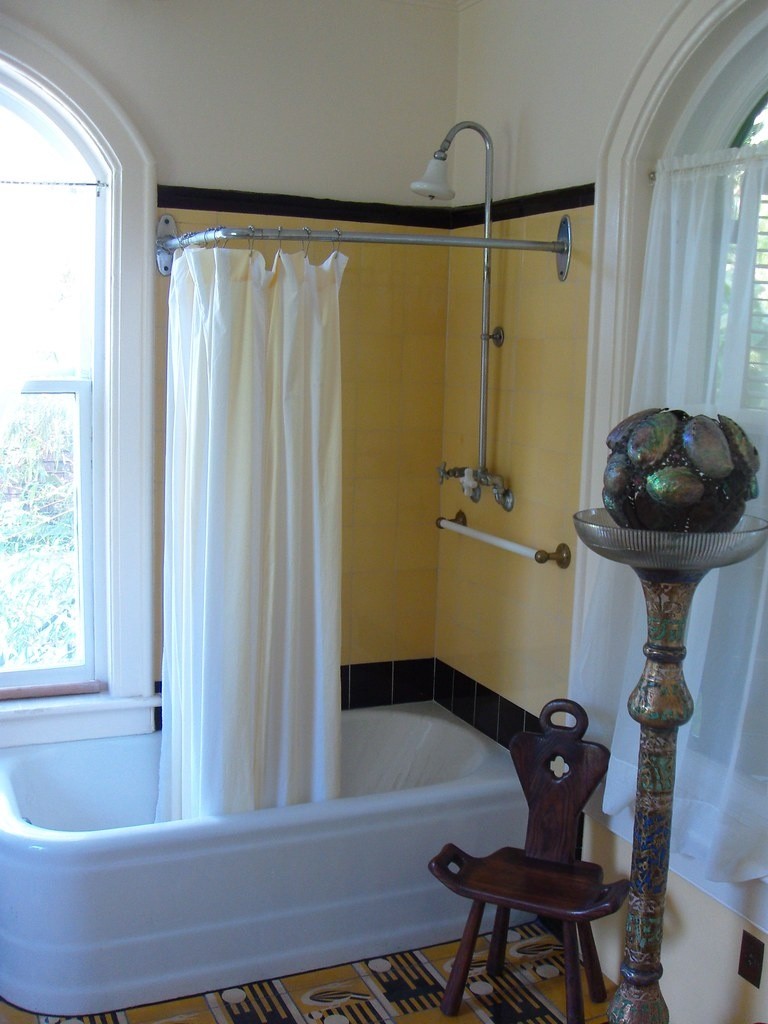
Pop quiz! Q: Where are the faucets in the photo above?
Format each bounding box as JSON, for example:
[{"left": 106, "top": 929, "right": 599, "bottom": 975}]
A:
[{"left": 459, "top": 469, "right": 478, "bottom": 497}]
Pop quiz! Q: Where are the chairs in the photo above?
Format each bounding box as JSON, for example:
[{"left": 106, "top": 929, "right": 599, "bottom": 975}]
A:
[{"left": 429, "top": 699, "right": 631, "bottom": 1024}]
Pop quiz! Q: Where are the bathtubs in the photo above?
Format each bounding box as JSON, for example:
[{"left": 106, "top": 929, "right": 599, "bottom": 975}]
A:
[{"left": 1, "top": 698, "right": 543, "bottom": 1018}]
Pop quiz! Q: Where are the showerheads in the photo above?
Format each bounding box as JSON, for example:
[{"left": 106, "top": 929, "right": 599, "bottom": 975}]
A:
[{"left": 409, "top": 158, "right": 455, "bottom": 201}]
